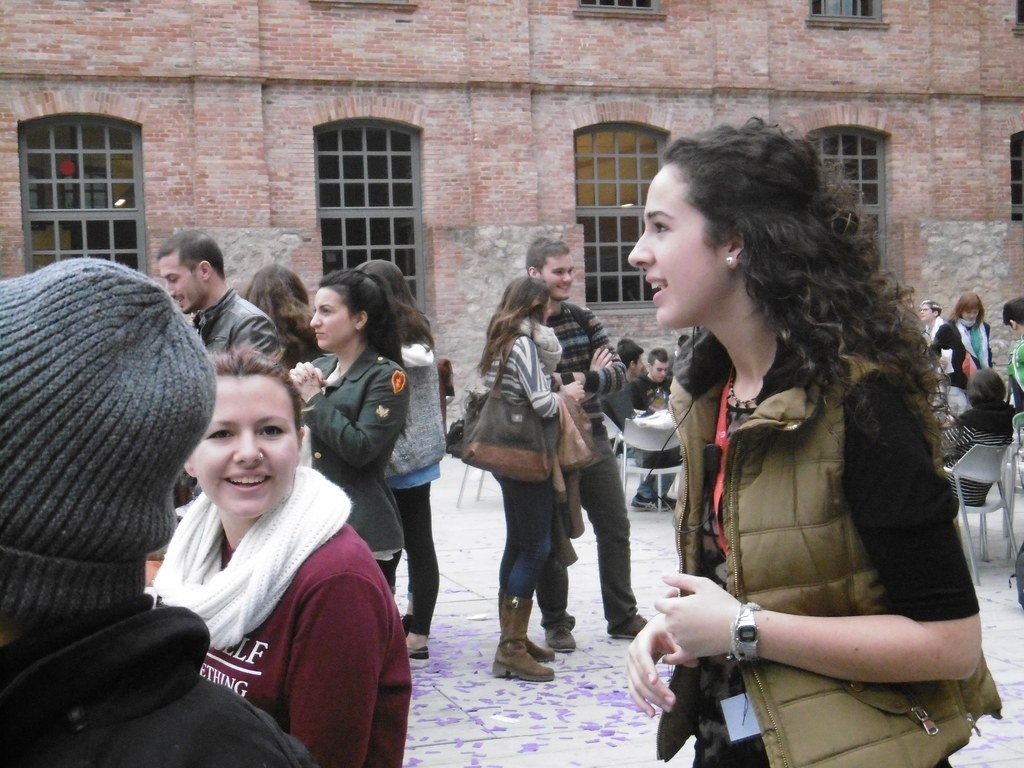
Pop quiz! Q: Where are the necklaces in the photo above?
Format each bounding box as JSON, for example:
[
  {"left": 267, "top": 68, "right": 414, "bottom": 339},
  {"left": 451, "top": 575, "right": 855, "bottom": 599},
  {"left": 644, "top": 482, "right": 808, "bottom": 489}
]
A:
[{"left": 725, "top": 373, "right": 759, "bottom": 420}]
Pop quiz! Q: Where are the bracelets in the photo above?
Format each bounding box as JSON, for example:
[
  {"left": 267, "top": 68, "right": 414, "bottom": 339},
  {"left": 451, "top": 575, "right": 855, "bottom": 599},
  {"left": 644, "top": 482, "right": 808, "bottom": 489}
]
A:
[{"left": 730, "top": 601, "right": 761, "bottom": 662}]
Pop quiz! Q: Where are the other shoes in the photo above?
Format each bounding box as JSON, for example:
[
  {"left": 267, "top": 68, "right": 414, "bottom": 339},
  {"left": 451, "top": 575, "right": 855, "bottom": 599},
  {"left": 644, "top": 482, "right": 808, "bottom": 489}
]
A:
[{"left": 411, "top": 647, "right": 430, "bottom": 664}]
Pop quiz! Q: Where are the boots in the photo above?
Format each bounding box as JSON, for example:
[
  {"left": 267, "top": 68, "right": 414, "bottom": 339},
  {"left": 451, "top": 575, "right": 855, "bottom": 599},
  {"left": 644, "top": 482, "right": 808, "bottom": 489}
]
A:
[
  {"left": 492, "top": 595, "right": 553, "bottom": 683},
  {"left": 498, "top": 590, "right": 556, "bottom": 662}
]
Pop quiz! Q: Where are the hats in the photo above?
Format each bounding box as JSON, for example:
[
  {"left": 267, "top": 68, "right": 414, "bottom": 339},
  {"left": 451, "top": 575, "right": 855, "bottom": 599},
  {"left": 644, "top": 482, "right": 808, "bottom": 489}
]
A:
[{"left": 1, "top": 257, "right": 217, "bottom": 620}]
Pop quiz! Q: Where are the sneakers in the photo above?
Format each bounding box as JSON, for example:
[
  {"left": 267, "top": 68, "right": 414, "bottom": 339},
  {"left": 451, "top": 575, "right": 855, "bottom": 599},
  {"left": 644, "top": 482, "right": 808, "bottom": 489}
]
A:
[
  {"left": 544, "top": 624, "right": 577, "bottom": 649},
  {"left": 632, "top": 492, "right": 677, "bottom": 510},
  {"left": 610, "top": 615, "right": 649, "bottom": 638}
]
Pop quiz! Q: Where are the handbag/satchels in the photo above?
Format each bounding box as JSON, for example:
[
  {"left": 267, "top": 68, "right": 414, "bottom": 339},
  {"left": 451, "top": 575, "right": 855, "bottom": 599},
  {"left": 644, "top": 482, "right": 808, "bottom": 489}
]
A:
[
  {"left": 460, "top": 335, "right": 551, "bottom": 483},
  {"left": 437, "top": 358, "right": 457, "bottom": 437}
]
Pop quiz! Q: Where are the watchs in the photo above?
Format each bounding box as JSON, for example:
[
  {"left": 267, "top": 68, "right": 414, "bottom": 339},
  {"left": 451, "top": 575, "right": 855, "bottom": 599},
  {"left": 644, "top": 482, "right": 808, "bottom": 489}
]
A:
[{"left": 736, "top": 610, "right": 760, "bottom": 658}]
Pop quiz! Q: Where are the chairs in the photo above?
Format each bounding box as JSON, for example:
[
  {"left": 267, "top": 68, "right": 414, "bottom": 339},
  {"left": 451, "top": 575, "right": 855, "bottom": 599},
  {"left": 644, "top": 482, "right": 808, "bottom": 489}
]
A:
[
  {"left": 621, "top": 417, "right": 682, "bottom": 514},
  {"left": 1002, "top": 434, "right": 1024, "bottom": 538},
  {"left": 599, "top": 412, "right": 636, "bottom": 470},
  {"left": 944, "top": 443, "right": 1018, "bottom": 586}
]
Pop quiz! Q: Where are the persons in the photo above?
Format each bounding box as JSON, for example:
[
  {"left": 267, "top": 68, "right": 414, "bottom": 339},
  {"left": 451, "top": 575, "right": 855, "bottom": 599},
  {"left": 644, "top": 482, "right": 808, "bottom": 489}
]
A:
[
  {"left": 940, "top": 365, "right": 1016, "bottom": 506},
  {"left": 919, "top": 300, "right": 947, "bottom": 346},
  {"left": 139, "top": 344, "right": 412, "bottom": 768},
  {"left": 156, "top": 230, "right": 288, "bottom": 503},
  {"left": 931, "top": 293, "right": 993, "bottom": 423},
  {"left": 601, "top": 338, "right": 676, "bottom": 512},
  {"left": 1003, "top": 296, "right": 1024, "bottom": 415},
  {"left": 672, "top": 333, "right": 691, "bottom": 364},
  {"left": 354, "top": 259, "right": 447, "bottom": 659},
  {"left": 283, "top": 270, "right": 410, "bottom": 597},
  {"left": 626, "top": 116, "right": 1002, "bottom": 768},
  {"left": 462, "top": 276, "right": 585, "bottom": 684},
  {"left": 246, "top": 264, "right": 324, "bottom": 368},
  {"left": 0, "top": 257, "right": 316, "bottom": 768},
  {"left": 526, "top": 235, "right": 647, "bottom": 654},
  {"left": 627, "top": 347, "right": 674, "bottom": 417}
]
